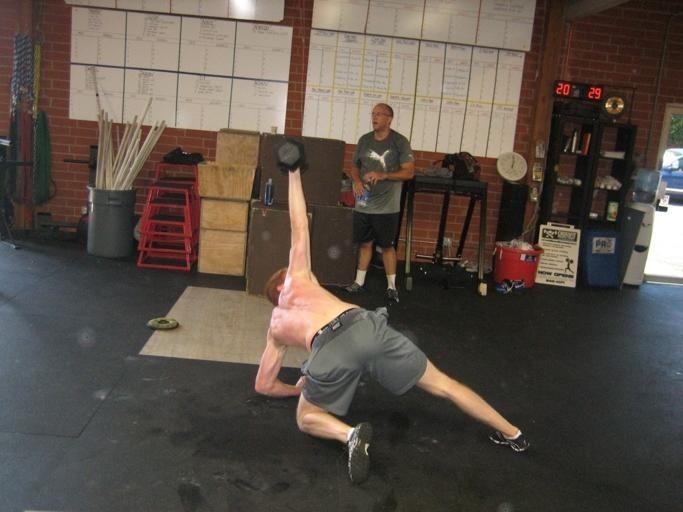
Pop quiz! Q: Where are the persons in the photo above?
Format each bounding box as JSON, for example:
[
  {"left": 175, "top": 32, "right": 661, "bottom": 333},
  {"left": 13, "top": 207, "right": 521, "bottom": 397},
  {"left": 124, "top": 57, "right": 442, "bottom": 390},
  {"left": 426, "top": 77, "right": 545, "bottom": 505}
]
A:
[
  {"left": 253, "top": 167, "right": 529, "bottom": 485},
  {"left": 338, "top": 104, "right": 415, "bottom": 303}
]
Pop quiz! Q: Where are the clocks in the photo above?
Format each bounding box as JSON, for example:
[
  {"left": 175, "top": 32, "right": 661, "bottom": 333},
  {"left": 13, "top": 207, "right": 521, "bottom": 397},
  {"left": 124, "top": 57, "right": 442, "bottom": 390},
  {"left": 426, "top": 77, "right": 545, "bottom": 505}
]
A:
[
  {"left": 496, "top": 151, "right": 528, "bottom": 182},
  {"left": 601, "top": 92, "right": 626, "bottom": 119}
]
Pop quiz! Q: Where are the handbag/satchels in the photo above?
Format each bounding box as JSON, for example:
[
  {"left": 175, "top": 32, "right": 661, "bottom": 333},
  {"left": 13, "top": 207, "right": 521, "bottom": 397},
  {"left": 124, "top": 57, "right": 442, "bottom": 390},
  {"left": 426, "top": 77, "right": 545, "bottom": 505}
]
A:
[
  {"left": 442, "top": 152, "right": 481, "bottom": 181},
  {"left": 164, "top": 148, "right": 203, "bottom": 165}
]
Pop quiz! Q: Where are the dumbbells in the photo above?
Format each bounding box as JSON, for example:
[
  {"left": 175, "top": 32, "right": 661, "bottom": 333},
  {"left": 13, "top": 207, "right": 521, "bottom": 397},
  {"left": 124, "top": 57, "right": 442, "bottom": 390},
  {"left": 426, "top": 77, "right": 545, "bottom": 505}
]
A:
[{"left": 277, "top": 136, "right": 307, "bottom": 173}]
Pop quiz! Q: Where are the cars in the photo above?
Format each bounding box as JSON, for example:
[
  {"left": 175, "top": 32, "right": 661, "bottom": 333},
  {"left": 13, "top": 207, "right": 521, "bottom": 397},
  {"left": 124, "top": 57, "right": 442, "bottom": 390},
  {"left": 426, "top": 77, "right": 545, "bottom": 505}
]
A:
[{"left": 661, "top": 148, "right": 683, "bottom": 199}]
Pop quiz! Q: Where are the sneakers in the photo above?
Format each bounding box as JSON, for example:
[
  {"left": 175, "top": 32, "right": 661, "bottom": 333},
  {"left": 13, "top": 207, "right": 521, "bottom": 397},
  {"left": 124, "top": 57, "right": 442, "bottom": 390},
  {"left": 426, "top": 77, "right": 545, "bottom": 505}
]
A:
[
  {"left": 385, "top": 288, "right": 400, "bottom": 303},
  {"left": 489, "top": 430, "right": 530, "bottom": 452},
  {"left": 345, "top": 282, "right": 367, "bottom": 295},
  {"left": 495, "top": 279, "right": 525, "bottom": 295},
  {"left": 348, "top": 422, "right": 374, "bottom": 484}
]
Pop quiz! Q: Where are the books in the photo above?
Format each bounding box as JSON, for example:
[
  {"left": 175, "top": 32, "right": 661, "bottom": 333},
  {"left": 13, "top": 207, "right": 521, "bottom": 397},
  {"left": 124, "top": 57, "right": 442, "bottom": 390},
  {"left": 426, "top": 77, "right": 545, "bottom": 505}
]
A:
[
  {"left": 598, "top": 150, "right": 625, "bottom": 160},
  {"left": 570, "top": 129, "right": 578, "bottom": 154},
  {"left": 580, "top": 133, "right": 591, "bottom": 155},
  {"left": 561, "top": 136, "right": 571, "bottom": 152}
]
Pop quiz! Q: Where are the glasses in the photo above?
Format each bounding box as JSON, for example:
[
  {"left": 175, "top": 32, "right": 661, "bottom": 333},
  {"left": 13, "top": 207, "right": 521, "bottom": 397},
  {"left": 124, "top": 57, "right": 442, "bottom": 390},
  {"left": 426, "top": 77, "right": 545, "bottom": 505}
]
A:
[{"left": 371, "top": 111, "right": 390, "bottom": 117}]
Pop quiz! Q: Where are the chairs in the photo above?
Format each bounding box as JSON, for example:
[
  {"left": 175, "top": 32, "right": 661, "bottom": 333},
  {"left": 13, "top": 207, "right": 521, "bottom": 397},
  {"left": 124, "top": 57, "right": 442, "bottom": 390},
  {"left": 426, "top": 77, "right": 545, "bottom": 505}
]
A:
[{"left": 137, "top": 163, "right": 201, "bottom": 271}]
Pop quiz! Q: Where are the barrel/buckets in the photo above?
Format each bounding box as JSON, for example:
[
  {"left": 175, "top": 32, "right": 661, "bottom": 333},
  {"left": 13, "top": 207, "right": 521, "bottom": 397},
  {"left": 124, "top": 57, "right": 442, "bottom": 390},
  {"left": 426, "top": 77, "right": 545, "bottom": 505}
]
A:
[
  {"left": 633, "top": 168, "right": 660, "bottom": 203},
  {"left": 494, "top": 243, "right": 543, "bottom": 288},
  {"left": 86, "top": 183, "right": 137, "bottom": 257}
]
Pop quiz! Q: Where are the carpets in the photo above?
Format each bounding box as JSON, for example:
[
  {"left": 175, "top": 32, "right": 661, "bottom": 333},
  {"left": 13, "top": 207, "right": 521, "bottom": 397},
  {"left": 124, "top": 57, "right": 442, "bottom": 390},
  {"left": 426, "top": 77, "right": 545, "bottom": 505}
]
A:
[{"left": 139, "top": 286, "right": 309, "bottom": 369}]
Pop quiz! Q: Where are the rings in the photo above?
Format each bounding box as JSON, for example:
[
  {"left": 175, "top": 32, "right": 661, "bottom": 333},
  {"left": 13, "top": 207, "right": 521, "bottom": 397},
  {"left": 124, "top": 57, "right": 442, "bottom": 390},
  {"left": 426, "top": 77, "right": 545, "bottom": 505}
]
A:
[{"left": 369, "top": 176, "right": 372, "bottom": 180}]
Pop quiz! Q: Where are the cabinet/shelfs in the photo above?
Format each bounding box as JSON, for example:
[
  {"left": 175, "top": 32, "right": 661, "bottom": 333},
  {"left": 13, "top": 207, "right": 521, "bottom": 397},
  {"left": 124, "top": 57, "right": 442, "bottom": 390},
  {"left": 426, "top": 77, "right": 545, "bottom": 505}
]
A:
[{"left": 533, "top": 113, "right": 638, "bottom": 274}]
